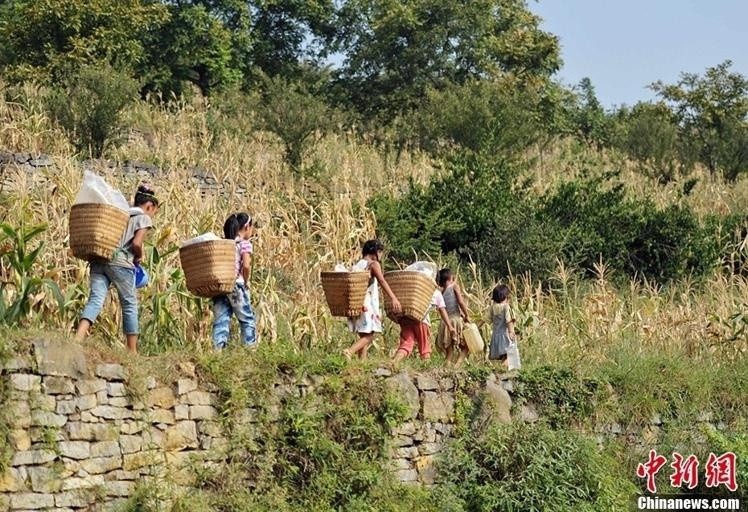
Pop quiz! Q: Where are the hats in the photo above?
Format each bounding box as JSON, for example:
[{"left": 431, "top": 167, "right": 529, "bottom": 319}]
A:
[{"left": 136, "top": 264, "right": 148, "bottom": 288}]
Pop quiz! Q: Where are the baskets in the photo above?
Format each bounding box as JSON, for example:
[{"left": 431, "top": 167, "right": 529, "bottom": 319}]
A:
[{"left": 69, "top": 203, "right": 130, "bottom": 263}]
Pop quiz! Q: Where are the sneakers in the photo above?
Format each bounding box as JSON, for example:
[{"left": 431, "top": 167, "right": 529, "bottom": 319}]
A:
[{"left": 343, "top": 349, "right": 353, "bottom": 360}]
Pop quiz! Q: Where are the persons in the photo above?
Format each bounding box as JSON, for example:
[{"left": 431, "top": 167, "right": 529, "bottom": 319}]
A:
[
  {"left": 391, "top": 266, "right": 459, "bottom": 364},
  {"left": 434, "top": 267, "right": 472, "bottom": 372},
  {"left": 210, "top": 210, "right": 257, "bottom": 355},
  {"left": 341, "top": 238, "right": 403, "bottom": 362},
  {"left": 474, "top": 283, "right": 515, "bottom": 369},
  {"left": 71, "top": 184, "right": 161, "bottom": 356}
]
